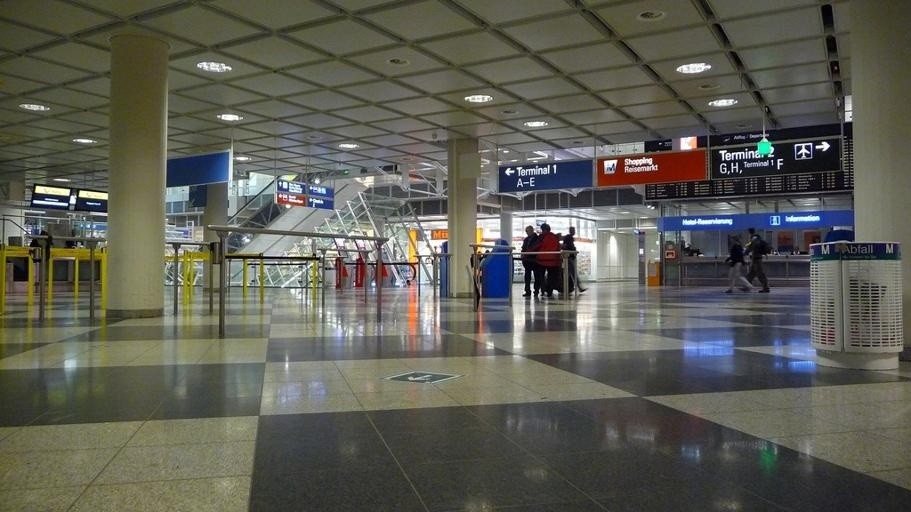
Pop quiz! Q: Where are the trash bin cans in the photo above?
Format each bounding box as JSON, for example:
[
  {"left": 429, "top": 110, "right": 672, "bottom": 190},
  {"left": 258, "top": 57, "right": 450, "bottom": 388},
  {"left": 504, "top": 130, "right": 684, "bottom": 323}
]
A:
[{"left": 810, "top": 240, "right": 904, "bottom": 371}]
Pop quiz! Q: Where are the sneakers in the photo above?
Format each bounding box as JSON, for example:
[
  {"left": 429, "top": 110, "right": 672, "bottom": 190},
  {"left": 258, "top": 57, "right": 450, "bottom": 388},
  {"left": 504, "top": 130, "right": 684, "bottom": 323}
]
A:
[
  {"left": 523, "top": 287, "right": 588, "bottom": 297},
  {"left": 723, "top": 287, "right": 771, "bottom": 294}
]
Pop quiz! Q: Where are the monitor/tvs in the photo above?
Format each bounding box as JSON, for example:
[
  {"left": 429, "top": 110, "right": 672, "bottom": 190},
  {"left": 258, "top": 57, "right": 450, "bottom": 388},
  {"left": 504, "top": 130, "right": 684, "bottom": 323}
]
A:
[
  {"left": 30, "top": 183, "right": 72, "bottom": 211},
  {"left": 73, "top": 188, "right": 108, "bottom": 213}
]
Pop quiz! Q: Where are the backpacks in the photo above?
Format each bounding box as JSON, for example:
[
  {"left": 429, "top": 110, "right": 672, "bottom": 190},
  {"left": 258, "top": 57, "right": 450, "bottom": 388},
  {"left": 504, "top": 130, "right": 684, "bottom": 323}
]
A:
[{"left": 751, "top": 234, "right": 773, "bottom": 256}]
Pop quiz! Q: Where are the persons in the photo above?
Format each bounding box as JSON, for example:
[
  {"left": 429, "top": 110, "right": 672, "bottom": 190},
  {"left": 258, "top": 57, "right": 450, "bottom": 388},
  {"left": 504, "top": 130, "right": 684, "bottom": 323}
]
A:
[
  {"left": 725, "top": 234, "right": 753, "bottom": 294},
  {"left": 742, "top": 228, "right": 771, "bottom": 293},
  {"left": 790, "top": 247, "right": 802, "bottom": 255},
  {"left": 520, "top": 223, "right": 588, "bottom": 297}
]
